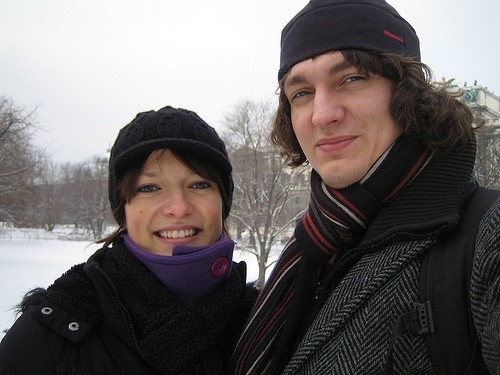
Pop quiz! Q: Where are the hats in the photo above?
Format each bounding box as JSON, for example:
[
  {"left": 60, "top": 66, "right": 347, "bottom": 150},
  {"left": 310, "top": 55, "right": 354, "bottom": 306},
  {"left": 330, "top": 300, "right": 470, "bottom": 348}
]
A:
[
  {"left": 278, "top": 0, "right": 422, "bottom": 81},
  {"left": 108, "top": 106, "right": 234, "bottom": 225}
]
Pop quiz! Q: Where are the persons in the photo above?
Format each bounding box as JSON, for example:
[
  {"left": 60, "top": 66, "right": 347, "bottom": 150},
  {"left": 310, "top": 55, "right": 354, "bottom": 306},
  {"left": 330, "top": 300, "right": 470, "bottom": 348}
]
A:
[
  {"left": 0, "top": 105, "right": 264, "bottom": 375},
  {"left": 225, "top": 0, "right": 500, "bottom": 375}
]
